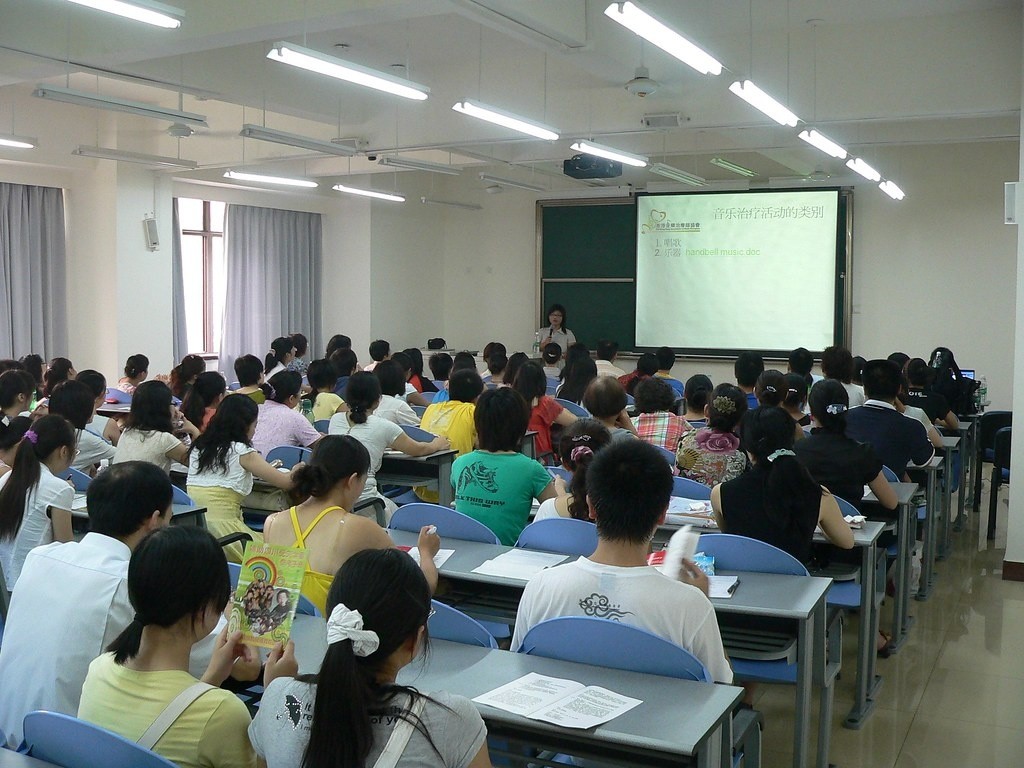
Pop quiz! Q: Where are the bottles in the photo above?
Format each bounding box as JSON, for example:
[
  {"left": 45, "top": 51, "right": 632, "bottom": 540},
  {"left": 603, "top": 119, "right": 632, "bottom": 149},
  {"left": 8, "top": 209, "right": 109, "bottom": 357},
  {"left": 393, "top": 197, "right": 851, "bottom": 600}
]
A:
[
  {"left": 28, "top": 388, "right": 37, "bottom": 412},
  {"left": 298, "top": 399, "right": 315, "bottom": 426},
  {"left": 532, "top": 331, "right": 540, "bottom": 353},
  {"left": 96, "top": 459, "right": 109, "bottom": 476}
]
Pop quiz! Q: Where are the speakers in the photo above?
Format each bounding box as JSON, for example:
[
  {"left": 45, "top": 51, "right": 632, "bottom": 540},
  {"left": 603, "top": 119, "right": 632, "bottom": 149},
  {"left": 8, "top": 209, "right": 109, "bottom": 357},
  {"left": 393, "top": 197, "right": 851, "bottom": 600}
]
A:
[
  {"left": 1004, "top": 181, "right": 1022, "bottom": 224},
  {"left": 330, "top": 137, "right": 364, "bottom": 151},
  {"left": 145, "top": 218, "right": 160, "bottom": 247},
  {"left": 644, "top": 112, "right": 683, "bottom": 127}
]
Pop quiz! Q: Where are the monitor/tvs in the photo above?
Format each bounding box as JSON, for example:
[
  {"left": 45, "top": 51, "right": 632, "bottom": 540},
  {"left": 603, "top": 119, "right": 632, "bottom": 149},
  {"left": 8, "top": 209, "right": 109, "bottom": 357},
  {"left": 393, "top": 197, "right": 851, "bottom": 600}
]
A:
[{"left": 951, "top": 369, "right": 975, "bottom": 381}]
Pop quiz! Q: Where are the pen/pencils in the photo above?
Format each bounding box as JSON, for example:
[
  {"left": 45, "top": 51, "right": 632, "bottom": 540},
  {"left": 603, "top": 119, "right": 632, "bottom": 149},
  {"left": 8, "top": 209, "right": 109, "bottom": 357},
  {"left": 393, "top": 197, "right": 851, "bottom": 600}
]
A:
[
  {"left": 67, "top": 474, "right": 72, "bottom": 480},
  {"left": 433, "top": 433, "right": 452, "bottom": 442},
  {"left": 547, "top": 469, "right": 556, "bottom": 479},
  {"left": 727, "top": 579, "right": 740, "bottom": 594}
]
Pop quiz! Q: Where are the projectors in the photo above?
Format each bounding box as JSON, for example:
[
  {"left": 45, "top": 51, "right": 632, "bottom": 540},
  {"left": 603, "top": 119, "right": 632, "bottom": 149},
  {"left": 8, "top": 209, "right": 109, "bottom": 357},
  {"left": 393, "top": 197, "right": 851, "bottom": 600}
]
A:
[{"left": 563, "top": 158, "right": 623, "bottom": 179}]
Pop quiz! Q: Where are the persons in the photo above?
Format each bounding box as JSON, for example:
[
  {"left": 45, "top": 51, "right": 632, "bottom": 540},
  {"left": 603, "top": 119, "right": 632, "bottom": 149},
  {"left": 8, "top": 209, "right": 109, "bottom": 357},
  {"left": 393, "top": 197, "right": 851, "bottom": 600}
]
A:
[
  {"left": 243, "top": 580, "right": 290, "bottom": 636},
  {"left": 508, "top": 438, "right": 754, "bottom": 768},
  {"left": 710, "top": 405, "right": 893, "bottom": 663},
  {"left": 78, "top": 524, "right": 299, "bottom": 768},
  {"left": 0, "top": 460, "right": 261, "bottom": 768},
  {"left": 248, "top": 547, "right": 495, "bottom": 768},
  {"left": 0, "top": 304, "right": 982, "bottom": 618}
]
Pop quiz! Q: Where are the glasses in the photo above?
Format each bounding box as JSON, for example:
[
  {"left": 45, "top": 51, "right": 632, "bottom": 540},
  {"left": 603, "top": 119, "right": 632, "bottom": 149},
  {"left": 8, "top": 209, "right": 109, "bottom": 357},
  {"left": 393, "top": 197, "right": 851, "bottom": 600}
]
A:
[
  {"left": 426, "top": 605, "right": 437, "bottom": 620},
  {"left": 74, "top": 449, "right": 80, "bottom": 456},
  {"left": 104, "top": 388, "right": 109, "bottom": 395},
  {"left": 292, "top": 348, "right": 298, "bottom": 352},
  {"left": 169, "top": 400, "right": 177, "bottom": 408},
  {"left": 549, "top": 313, "right": 562, "bottom": 318}
]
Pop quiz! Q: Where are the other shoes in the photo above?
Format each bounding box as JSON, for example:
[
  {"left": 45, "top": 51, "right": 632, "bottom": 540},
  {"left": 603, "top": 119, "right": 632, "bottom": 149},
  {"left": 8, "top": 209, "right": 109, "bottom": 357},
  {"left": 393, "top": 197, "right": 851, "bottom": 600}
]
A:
[{"left": 878, "top": 630, "right": 893, "bottom": 658}]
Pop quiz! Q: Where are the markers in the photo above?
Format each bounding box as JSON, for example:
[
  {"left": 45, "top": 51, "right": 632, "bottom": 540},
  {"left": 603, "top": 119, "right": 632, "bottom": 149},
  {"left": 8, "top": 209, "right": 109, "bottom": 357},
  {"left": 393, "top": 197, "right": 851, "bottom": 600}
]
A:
[{"left": 427, "top": 527, "right": 437, "bottom": 535}]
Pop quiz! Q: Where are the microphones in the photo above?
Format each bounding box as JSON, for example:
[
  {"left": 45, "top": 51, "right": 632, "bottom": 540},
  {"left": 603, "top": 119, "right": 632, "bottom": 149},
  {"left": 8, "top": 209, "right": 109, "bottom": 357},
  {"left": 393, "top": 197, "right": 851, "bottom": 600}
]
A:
[{"left": 549, "top": 324, "right": 555, "bottom": 338}]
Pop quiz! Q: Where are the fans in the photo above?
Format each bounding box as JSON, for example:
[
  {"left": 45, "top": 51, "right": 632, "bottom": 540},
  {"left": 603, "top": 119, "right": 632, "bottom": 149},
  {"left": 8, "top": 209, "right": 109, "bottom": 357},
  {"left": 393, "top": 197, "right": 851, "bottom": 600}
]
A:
[
  {"left": 767, "top": 170, "right": 848, "bottom": 183},
  {"left": 578, "top": 38, "right": 662, "bottom": 99},
  {"left": 468, "top": 183, "right": 503, "bottom": 195},
  {"left": 101, "top": 54, "right": 242, "bottom": 140}
]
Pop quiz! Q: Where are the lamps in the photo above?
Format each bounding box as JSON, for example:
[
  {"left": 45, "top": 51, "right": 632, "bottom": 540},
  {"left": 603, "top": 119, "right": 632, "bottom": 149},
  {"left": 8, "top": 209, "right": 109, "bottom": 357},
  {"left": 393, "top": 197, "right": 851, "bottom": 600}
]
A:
[
  {"left": 845, "top": 122, "right": 881, "bottom": 182},
  {"left": 70, "top": 74, "right": 200, "bottom": 170},
  {"left": 32, "top": 10, "right": 210, "bottom": 130},
  {"left": 603, "top": 0, "right": 734, "bottom": 78},
  {"left": 648, "top": 132, "right": 708, "bottom": 188},
  {"left": 728, "top": 0, "right": 806, "bottom": 128},
  {"left": 568, "top": 87, "right": 650, "bottom": 168},
  {"left": 0, "top": 93, "right": 41, "bottom": 149},
  {"left": 378, "top": 103, "right": 464, "bottom": 177},
  {"left": 796, "top": 18, "right": 847, "bottom": 161},
  {"left": 265, "top": 0, "right": 431, "bottom": 101},
  {"left": 238, "top": 99, "right": 358, "bottom": 159},
  {"left": 420, "top": 172, "right": 486, "bottom": 211},
  {"left": 221, "top": 137, "right": 318, "bottom": 188},
  {"left": 330, "top": 156, "right": 406, "bottom": 204},
  {"left": 66, "top": 0, "right": 186, "bottom": 30},
  {"left": 477, "top": 146, "right": 548, "bottom": 195},
  {"left": 878, "top": 146, "right": 906, "bottom": 201},
  {"left": 710, "top": 157, "right": 759, "bottom": 178},
  {"left": 452, "top": 21, "right": 562, "bottom": 144}
]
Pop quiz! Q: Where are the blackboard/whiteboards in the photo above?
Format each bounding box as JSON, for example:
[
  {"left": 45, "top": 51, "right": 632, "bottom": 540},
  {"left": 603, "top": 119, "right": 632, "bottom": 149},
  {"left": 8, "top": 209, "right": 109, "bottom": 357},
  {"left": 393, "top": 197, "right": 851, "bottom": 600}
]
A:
[{"left": 535, "top": 185, "right": 855, "bottom": 365}]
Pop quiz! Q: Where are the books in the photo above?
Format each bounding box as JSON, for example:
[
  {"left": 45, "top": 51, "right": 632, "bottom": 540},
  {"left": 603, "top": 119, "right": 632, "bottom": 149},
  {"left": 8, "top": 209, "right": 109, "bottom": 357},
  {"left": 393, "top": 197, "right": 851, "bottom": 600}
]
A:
[
  {"left": 227, "top": 541, "right": 309, "bottom": 651},
  {"left": 646, "top": 525, "right": 700, "bottom": 582}
]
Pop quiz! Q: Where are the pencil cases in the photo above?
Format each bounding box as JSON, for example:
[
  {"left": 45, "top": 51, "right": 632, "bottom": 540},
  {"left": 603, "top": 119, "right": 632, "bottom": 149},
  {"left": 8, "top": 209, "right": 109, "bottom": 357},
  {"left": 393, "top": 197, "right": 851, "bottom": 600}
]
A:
[{"left": 104, "top": 398, "right": 119, "bottom": 404}]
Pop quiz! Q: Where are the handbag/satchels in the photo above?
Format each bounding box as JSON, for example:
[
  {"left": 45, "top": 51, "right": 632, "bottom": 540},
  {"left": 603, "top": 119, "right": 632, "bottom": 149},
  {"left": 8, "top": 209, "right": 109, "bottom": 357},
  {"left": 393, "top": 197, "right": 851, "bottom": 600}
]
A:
[
  {"left": 427, "top": 337, "right": 446, "bottom": 350},
  {"left": 952, "top": 377, "right": 982, "bottom": 414}
]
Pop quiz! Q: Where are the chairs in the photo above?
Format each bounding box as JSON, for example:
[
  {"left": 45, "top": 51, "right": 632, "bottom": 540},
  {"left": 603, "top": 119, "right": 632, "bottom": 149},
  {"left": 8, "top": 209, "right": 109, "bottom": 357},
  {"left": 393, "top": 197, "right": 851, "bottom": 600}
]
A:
[
  {"left": 986, "top": 427, "right": 1011, "bottom": 539},
  {"left": 689, "top": 421, "right": 706, "bottom": 429},
  {"left": 694, "top": 533, "right": 845, "bottom": 768},
  {"left": 428, "top": 599, "right": 499, "bottom": 649},
  {"left": 543, "top": 465, "right": 573, "bottom": 493},
  {"left": 671, "top": 476, "right": 714, "bottom": 501},
  {"left": 652, "top": 444, "right": 677, "bottom": 466},
  {"left": 388, "top": 502, "right": 511, "bottom": 639},
  {"left": 15, "top": 710, "right": 181, "bottom": 768},
  {"left": 228, "top": 562, "right": 323, "bottom": 618},
  {"left": 0, "top": 403, "right": 980, "bottom": 768},
  {"left": 56, "top": 467, "right": 93, "bottom": 491},
  {"left": 106, "top": 373, "right": 686, "bottom": 419},
  {"left": 882, "top": 466, "right": 916, "bottom": 633},
  {"left": 313, "top": 420, "right": 330, "bottom": 435},
  {"left": 516, "top": 616, "right": 765, "bottom": 768},
  {"left": 391, "top": 424, "right": 439, "bottom": 505},
  {"left": 377, "top": 482, "right": 407, "bottom": 498},
  {"left": 951, "top": 452, "right": 968, "bottom": 534},
  {"left": 514, "top": 517, "right": 599, "bottom": 556},
  {"left": 171, "top": 484, "right": 254, "bottom": 556},
  {"left": 973, "top": 411, "right": 1013, "bottom": 512},
  {"left": 410, "top": 405, "right": 427, "bottom": 420},
  {"left": 917, "top": 506, "right": 935, "bottom": 602},
  {"left": 243, "top": 446, "right": 313, "bottom": 529},
  {"left": 825, "top": 495, "right": 885, "bottom": 702}
]
